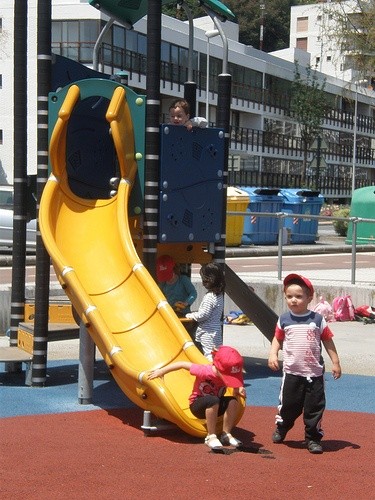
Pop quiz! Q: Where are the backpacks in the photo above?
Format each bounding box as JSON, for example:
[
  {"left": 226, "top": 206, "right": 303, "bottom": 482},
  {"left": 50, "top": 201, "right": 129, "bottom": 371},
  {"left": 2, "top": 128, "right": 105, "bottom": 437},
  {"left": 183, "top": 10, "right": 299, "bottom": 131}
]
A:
[
  {"left": 355, "top": 304, "right": 375, "bottom": 323},
  {"left": 313, "top": 296, "right": 333, "bottom": 320},
  {"left": 332, "top": 295, "right": 355, "bottom": 322}
]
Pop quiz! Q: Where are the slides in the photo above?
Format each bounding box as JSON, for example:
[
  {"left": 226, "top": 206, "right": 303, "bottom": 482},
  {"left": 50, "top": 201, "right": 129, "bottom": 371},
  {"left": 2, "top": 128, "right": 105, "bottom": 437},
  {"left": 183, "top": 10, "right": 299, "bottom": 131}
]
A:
[{"left": 36, "top": 176, "right": 247, "bottom": 438}]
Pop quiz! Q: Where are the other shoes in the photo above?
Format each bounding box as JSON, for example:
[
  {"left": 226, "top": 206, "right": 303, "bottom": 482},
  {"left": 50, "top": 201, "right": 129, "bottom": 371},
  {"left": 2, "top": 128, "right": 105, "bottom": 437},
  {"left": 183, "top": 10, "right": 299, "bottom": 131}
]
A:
[
  {"left": 205, "top": 434, "right": 223, "bottom": 450},
  {"left": 220, "top": 434, "right": 243, "bottom": 446}
]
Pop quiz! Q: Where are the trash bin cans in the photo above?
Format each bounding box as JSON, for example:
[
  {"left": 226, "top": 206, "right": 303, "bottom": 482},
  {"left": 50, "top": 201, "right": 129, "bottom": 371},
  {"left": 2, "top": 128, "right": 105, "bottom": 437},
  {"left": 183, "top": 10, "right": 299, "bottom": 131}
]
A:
[
  {"left": 225, "top": 187, "right": 251, "bottom": 247},
  {"left": 281, "top": 188, "right": 325, "bottom": 245},
  {"left": 239, "top": 186, "right": 283, "bottom": 244},
  {"left": 345, "top": 186, "right": 375, "bottom": 243}
]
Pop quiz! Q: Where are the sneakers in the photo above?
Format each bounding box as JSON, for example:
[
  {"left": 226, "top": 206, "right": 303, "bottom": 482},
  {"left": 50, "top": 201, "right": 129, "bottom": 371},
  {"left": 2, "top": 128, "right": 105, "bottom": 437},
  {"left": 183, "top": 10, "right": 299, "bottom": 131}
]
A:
[
  {"left": 305, "top": 439, "right": 323, "bottom": 453},
  {"left": 271, "top": 424, "right": 294, "bottom": 442}
]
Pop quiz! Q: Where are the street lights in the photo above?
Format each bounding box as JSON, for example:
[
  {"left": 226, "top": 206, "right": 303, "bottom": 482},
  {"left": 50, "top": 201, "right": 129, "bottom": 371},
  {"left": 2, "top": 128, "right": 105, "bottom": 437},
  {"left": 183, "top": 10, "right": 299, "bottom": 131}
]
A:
[
  {"left": 204, "top": 29, "right": 221, "bottom": 127},
  {"left": 352, "top": 79, "right": 369, "bottom": 197}
]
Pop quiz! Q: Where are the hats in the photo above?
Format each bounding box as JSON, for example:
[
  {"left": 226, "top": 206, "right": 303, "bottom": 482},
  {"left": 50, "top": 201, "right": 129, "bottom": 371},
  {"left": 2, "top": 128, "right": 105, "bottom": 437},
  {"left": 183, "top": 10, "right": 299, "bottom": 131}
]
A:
[
  {"left": 283, "top": 273, "right": 315, "bottom": 295},
  {"left": 213, "top": 346, "right": 244, "bottom": 387},
  {"left": 155, "top": 255, "right": 175, "bottom": 281}
]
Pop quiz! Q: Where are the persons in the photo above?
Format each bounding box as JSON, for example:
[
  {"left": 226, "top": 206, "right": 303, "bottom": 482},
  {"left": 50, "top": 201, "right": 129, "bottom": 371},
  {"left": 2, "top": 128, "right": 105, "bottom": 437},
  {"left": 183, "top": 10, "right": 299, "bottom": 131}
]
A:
[
  {"left": 155, "top": 255, "right": 198, "bottom": 318},
  {"left": 185, "top": 263, "right": 225, "bottom": 362},
  {"left": 147, "top": 346, "right": 247, "bottom": 449},
  {"left": 268, "top": 273, "right": 341, "bottom": 454},
  {"left": 169, "top": 99, "right": 208, "bottom": 130}
]
